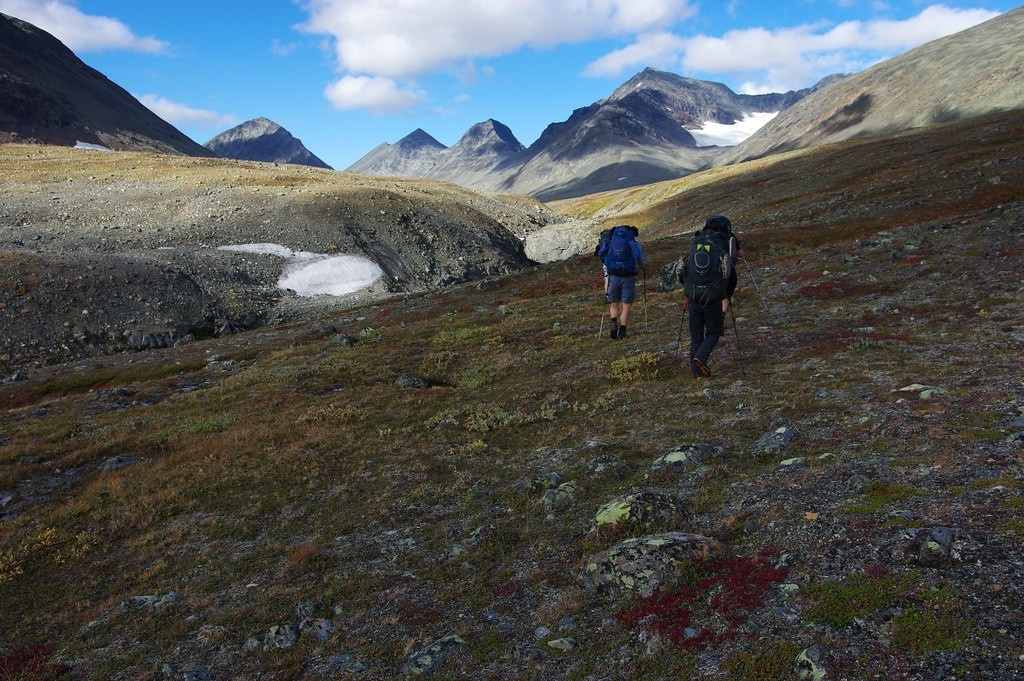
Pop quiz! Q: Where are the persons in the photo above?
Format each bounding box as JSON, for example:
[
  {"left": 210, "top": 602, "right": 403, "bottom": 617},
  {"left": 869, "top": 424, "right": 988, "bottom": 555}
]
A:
[
  {"left": 594, "top": 224, "right": 647, "bottom": 338},
  {"left": 679, "top": 213, "right": 744, "bottom": 380}
]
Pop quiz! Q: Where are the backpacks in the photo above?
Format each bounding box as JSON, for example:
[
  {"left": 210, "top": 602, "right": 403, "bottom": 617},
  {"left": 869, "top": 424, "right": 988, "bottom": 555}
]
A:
[
  {"left": 594, "top": 225, "right": 638, "bottom": 276},
  {"left": 674, "top": 215, "right": 740, "bottom": 308}
]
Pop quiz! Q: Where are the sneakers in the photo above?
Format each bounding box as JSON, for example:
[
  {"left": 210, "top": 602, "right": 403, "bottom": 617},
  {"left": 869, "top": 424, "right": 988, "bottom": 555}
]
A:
[
  {"left": 610, "top": 330, "right": 617, "bottom": 340},
  {"left": 618, "top": 332, "right": 630, "bottom": 339},
  {"left": 691, "top": 358, "right": 711, "bottom": 379}
]
